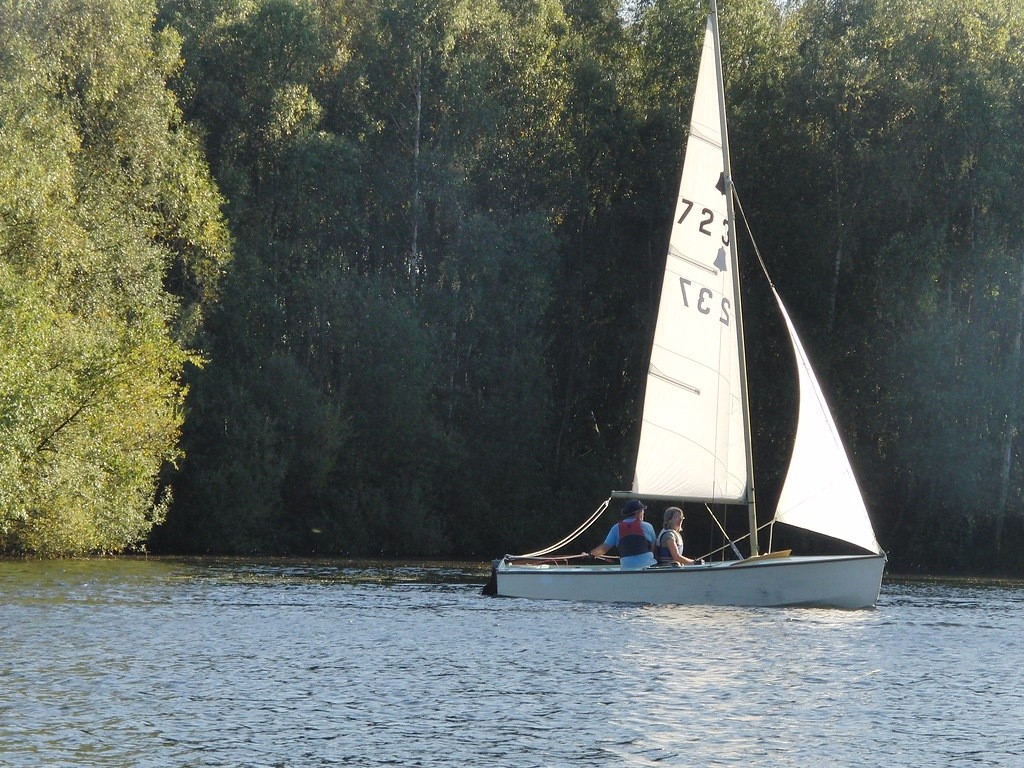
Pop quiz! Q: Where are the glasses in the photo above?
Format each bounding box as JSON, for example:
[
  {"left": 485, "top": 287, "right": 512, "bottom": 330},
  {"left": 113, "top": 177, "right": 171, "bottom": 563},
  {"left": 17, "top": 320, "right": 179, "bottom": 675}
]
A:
[{"left": 672, "top": 516, "right": 685, "bottom": 520}]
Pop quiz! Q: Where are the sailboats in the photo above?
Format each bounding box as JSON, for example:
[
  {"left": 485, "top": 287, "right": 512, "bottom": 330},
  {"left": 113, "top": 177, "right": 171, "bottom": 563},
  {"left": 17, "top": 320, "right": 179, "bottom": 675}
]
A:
[{"left": 480, "top": 1, "right": 887, "bottom": 607}]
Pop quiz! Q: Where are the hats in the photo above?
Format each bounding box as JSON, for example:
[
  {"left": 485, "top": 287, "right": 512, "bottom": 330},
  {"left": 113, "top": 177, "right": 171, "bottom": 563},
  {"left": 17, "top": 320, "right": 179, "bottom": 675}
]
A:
[{"left": 621, "top": 499, "right": 647, "bottom": 516}]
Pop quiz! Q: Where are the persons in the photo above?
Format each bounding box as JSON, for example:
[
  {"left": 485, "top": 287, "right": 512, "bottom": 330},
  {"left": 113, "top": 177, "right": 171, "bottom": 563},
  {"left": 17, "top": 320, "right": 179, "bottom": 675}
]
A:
[
  {"left": 590, "top": 500, "right": 658, "bottom": 568},
  {"left": 655, "top": 507, "right": 705, "bottom": 566}
]
